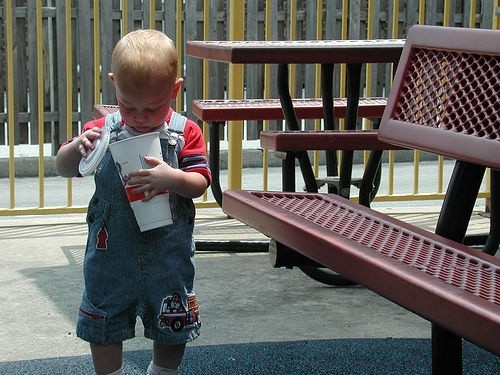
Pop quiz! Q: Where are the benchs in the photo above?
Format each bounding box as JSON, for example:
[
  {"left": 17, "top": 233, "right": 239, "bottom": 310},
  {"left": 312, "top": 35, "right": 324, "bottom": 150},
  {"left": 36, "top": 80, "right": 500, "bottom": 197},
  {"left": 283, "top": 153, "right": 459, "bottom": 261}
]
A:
[
  {"left": 221, "top": 23, "right": 499, "bottom": 375},
  {"left": 259, "top": 129, "right": 500, "bottom": 288},
  {"left": 191, "top": 96, "right": 388, "bottom": 211}
]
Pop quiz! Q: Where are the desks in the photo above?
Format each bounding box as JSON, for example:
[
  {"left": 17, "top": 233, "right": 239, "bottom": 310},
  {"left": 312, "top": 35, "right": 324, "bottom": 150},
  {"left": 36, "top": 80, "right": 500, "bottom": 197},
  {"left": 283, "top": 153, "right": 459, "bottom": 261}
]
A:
[{"left": 185, "top": 39, "right": 500, "bottom": 286}]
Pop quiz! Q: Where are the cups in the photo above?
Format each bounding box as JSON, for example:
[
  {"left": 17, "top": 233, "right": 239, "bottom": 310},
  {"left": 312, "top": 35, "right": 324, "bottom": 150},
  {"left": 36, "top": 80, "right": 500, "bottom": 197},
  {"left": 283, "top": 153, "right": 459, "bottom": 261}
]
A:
[{"left": 109, "top": 132, "right": 173, "bottom": 232}]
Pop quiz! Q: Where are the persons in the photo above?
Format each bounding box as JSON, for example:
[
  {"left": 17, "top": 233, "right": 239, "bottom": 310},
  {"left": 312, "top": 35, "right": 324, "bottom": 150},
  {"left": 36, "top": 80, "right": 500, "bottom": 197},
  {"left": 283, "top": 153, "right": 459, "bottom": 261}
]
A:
[{"left": 54, "top": 29, "right": 213, "bottom": 375}]
